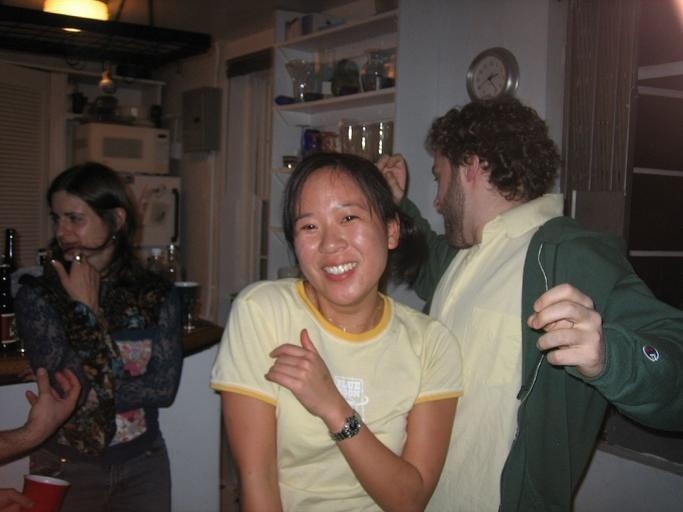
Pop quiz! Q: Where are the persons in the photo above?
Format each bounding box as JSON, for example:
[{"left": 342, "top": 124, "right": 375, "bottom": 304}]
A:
[
  {"left": 374, "top": 94, "right": 683, "bottom": 511},
  {"left": 15, "top": 160, "right": 184, "bottom": 511},
  {"left": 209, "top": 149, "right": 464, "bottom": 512},
  {"left": 0, "top": 366, "right": 81, "bottom": 512}
]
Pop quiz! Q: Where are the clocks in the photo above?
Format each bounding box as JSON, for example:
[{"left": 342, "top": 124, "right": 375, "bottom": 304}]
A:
[{"left": 464, "top": 47, "right": 519, "bottom": 104}]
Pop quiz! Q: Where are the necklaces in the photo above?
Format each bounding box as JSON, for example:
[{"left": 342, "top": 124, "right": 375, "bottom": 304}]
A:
[{"left": 312, "top": 293, "right": 382, "bottom": 333}]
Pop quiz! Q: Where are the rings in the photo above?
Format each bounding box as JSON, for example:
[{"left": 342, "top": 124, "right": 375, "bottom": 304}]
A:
[{"left": 72, "top": 255, "right": 81, "bottom": 265}]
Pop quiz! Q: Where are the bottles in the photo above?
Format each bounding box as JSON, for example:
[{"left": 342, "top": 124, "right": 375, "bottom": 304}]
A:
[
  {"left": 149, "top": 244, "right": 177, "bottom": 283},
  {"left": 289, "top": 49, "right": 389, "bottom": 101},
  {"left": 0, "top": 228, "right": 17, "bottom": 352},
  {"left": 303, "top": 120, "right": 391, "bottom": 163},
  {"left": 37, "top": 248, "right": 47, "bottom": 264}
]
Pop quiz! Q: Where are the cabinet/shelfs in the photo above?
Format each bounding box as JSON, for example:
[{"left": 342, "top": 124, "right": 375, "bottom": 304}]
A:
[{"left": 268, "top": 1, "right": 398, "bottom": 281}]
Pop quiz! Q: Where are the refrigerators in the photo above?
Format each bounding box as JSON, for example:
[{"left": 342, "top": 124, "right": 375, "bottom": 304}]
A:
[{"left": 124, "top": 176, "right": 183, "bottom": 274}]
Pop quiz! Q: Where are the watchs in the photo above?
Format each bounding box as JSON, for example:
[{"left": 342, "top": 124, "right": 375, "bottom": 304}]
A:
[{"left": 330, "top": 410, "right": 362, "bottom": 443}]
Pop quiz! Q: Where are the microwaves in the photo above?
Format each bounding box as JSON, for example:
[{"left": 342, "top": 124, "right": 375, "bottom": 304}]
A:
[{"left": 74, "top": 123, "right": 171, "bottom": 175}]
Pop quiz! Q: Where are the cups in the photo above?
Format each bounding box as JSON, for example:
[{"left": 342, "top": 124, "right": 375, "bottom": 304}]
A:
[
  {"left": 282, "top": 156, "right": 296, "bottom": 169},
  {"left": 176, "top": 281, "right": 200, "bottom": 331},
  {"left": 21, "top": 474, "right": 72, "bottom": 512}
]
